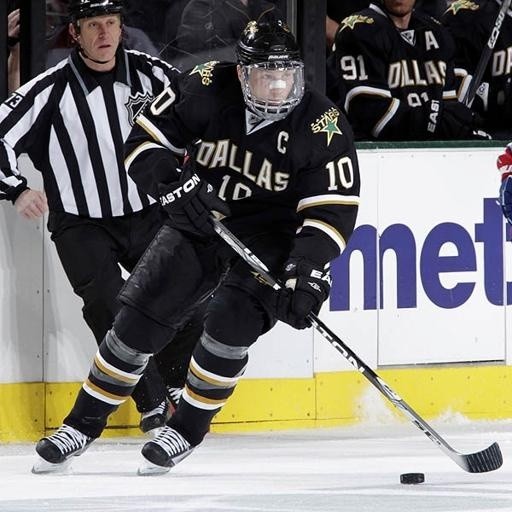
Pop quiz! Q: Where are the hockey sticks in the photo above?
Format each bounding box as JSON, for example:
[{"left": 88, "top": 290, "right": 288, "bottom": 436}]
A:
[{"left": 212, "top": 218, "right": 502, "bottom": 473}]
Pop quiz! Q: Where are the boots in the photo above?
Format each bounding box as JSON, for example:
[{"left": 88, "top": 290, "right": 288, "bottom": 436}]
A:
[
  {"left": 35, "top": 423, "right": 95, "bottom": 463},
  {"left": 140, "top": 385, "right": 210, "bottom": 466}
]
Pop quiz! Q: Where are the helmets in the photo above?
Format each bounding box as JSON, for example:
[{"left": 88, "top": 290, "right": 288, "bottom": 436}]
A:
[
  {"left": 67, "top": 1, "right": 121, "bottom": 23},
  {"left": 236, "top": 21, "right": 305, "bottom": 123}
]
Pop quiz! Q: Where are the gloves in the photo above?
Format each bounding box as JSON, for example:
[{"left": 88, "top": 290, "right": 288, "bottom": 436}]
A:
[
  {"left": 275, "top": 260, "right": 331, "bottom": 330},
  {"left": 421, "top": 99, "right": 474, "bottom": 140},
  {"left": 159, "top": 168, "right": 230, "bottom": 237}
]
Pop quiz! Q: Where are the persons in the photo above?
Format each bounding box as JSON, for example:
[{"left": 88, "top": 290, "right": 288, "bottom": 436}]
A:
[
  {"left": 0, "top": 0, "right": 203, "bottom": 433},
  {"left": 35, "top": 18, "right": 360, "bottom": 466},
  {"left": 496, "top": 140, "right": 512, "bottom": 226}
]
